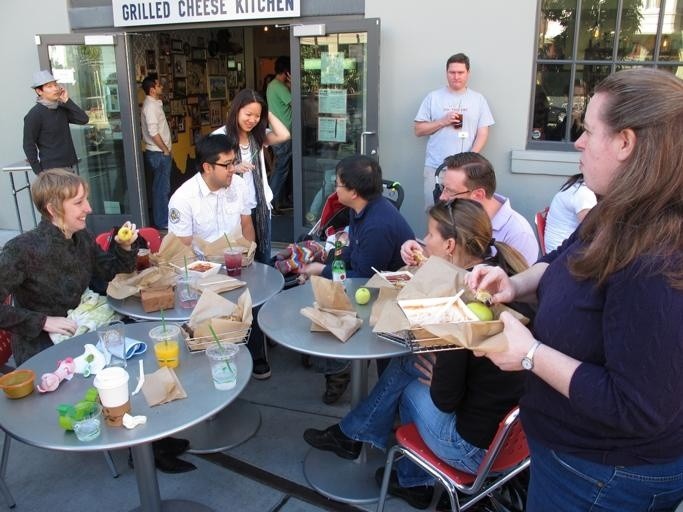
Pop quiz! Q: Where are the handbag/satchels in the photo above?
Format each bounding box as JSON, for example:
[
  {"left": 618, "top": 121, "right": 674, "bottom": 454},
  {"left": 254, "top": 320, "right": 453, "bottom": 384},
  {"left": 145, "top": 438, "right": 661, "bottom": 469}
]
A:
[{"left": 436, "top": 474, "right": 527, "bottom": 512}]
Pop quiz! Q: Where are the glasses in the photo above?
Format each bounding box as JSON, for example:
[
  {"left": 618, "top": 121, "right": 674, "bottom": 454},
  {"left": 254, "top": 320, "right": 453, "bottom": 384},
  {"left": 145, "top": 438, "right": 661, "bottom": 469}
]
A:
[
  {"left": 214, "top": 158, "right": 240, "bottom": 171},
  {"left": 439, "top": 184, "right": 472, "bottom": 197},
  {"left": 442, "top": 196, "right": 458, "bottom": 239}
]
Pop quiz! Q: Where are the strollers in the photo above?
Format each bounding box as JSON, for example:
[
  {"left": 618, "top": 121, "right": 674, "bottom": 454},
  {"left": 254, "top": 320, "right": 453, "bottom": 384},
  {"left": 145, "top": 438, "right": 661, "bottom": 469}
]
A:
[{"left": 282, "top": 177, "right": 405, "bottom": 290}]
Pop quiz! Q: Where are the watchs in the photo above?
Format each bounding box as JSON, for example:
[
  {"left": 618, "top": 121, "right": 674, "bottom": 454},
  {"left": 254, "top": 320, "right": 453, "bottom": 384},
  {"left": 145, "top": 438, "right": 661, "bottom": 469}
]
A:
[{"left": 521, "top": 340, "right": 541, "bottom": 371}]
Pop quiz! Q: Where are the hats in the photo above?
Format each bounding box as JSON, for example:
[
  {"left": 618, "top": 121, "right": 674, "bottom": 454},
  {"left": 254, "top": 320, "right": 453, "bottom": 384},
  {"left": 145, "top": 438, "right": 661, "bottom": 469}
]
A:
[{"left": 30, "top": 70, "right": 60, "bottom": 89}]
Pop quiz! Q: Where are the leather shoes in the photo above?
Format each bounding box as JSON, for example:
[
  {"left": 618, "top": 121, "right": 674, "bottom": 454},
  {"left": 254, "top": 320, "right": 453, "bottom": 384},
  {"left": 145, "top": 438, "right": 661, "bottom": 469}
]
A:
[
  {"left": 128, "top": 436, "right": 190, "bottom": 456},
  {"left": 127, "top": 450, "right": 197, "bottom": 473}
]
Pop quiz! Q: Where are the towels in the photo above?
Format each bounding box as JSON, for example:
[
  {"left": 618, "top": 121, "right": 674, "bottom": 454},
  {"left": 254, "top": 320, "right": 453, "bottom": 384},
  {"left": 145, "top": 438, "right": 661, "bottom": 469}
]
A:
[{"left": 97, "top": 336, "right": 147, "bottom": 359}]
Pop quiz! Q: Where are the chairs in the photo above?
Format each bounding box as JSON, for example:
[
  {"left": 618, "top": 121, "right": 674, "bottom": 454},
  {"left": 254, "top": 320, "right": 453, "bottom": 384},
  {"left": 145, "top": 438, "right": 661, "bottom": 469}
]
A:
[
  {"left": 95, "top": 228, "right": 160, "bottom": 253},
  {"left": 535, "top": 206, "right": 550, "bottom": 255},
  {"left": 377, "top": 406, "right": 532, "bottom": 512}
]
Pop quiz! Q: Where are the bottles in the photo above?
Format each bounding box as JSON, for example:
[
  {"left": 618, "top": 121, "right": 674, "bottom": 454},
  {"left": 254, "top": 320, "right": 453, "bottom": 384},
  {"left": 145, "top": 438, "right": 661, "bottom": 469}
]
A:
[
  {"left": 136, "top": 248, "right": 150, "bottom": 272},
  {"left": 332, "top": 240, "right": 347, "bottom": 294}
]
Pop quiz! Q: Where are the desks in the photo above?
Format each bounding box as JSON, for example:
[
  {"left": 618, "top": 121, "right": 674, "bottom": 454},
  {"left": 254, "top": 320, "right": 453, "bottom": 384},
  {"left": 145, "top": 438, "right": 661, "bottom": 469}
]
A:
[
  {"left": 1, "top": 157, "right": 83, "bottom": 234},
  {"left": 88, "top": 151, "right": 112, "bottom": 200}
]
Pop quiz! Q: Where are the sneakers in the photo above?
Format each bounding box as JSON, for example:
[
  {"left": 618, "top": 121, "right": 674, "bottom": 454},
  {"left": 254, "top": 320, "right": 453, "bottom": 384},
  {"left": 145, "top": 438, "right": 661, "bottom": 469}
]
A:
[
  {"left": 270, "top": 202, "right": 293, "bottom": 216},
  {"left": 156, "top": 222, "right": 168, "bottom": 230},
  {"left": 303, "top": 422, "right": 363, "bottom": 460},
  {"left": 375, "top": 466, "right": 434, "bottom": 510},
  {"left": 320, "top": 357, "right": 372, "bottom": 405},
  {"left": 251, "top": 357, "right": 272, "bottom": 380}
]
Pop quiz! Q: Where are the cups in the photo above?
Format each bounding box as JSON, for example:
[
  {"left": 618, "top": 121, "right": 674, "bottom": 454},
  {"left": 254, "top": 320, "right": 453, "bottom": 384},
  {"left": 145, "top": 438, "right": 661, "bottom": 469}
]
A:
[
  {"left": 174, "top": 272, "right": 199, "bottom": 308},
  {"left": 93, "top": 368, "right": 132, "bottom": 428},
  {"left": 66, "top": 402, "right": 102, "bottom": 441},
  {"left": 223, "top": 247, "right": 244, "bottom": 277},
  {"left": 454, "top": 114, "right": 463, "bottom": 129},
  {"left": 206, "top": 342, "right": 239, "bottom": 391},
  {"left": 148, "top": 325, "right": 180, "bottom": 370},
  {"left": 95, "top": 321, "right": 129, "bottom": 370}
]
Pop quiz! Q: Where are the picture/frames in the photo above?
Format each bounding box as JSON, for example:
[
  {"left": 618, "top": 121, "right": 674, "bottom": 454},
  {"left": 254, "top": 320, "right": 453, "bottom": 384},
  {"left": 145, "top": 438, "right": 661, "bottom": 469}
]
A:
[{"left": 145, "top": 34, "right": 237, "bottom": 146}]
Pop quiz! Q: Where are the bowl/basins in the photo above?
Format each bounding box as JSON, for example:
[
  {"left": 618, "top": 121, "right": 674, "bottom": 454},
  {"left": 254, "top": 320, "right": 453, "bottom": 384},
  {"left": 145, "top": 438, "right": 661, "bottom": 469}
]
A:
[
  {"left": 1, "top": 368, "right": 36, "bottom": 400},
  {"left": 180, "top": 260, "right": 222, "bottom": 279},
  {"left": 381, "top": 271, "right": 414, "bottom": 286},
  {"left": 397, "top": 294, "right": 480, "bottom": 349}
]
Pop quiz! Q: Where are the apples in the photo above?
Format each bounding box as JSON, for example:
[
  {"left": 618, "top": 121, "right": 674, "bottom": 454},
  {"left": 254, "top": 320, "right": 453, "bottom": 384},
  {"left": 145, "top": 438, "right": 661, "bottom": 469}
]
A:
[
  {"left": 118, "top": 227, "right": 132, "bottom": 242},
  {"left": 466, "top": 302, "right": 493, "bottom": 321},
  {"left": 355, "top": 288, "right": 371, "bottom": 304}
]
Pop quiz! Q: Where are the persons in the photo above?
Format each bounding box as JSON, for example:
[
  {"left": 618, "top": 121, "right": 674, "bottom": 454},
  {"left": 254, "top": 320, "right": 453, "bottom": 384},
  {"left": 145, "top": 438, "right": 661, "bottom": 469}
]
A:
[
  {"left": 1, "top": 168, "right": 197, "bottom": 475},
  {"left": 552, "top": 78, "right": 590, "bottom": 143},
  {"left": 465, "top": 67, "right": 683, "bottom": 512},
  {"left": 302, "top": 200, "right": 539, "bottom": 510},
  {"left": 532, "top": 83, "right": 550, "bottom": 141},
  {"left": 113, "top": 125, "right": 128, "bottom": 214},
  {"left": 209, "top": 89, "right": 291, "bottom": 265},
  {"left": 400, "top": 151, "right": 539, "bottom": 269},
  {"left": 23, "top": 70, "right": 89, "bottom": 176},
  {"left": 166, "top": 133, "right": 272, "bottom": 381},
  {"left": 266, "top": 56, "right": 293, "bottom": 216},
  {"left": 258, "top": 75, "right": 275, "bottom": 102},
  {"left": 413, "top": 53, "right": 496, "bottom": 216},
  {"left": 544, "top": 173, "right": 599, "bottom": 255},
  {"left": 140, "top": 72, "right": 172, "bottom": 231},
  {"left": 295, "top": 153, "right": 415, "bottom": 405}
]
